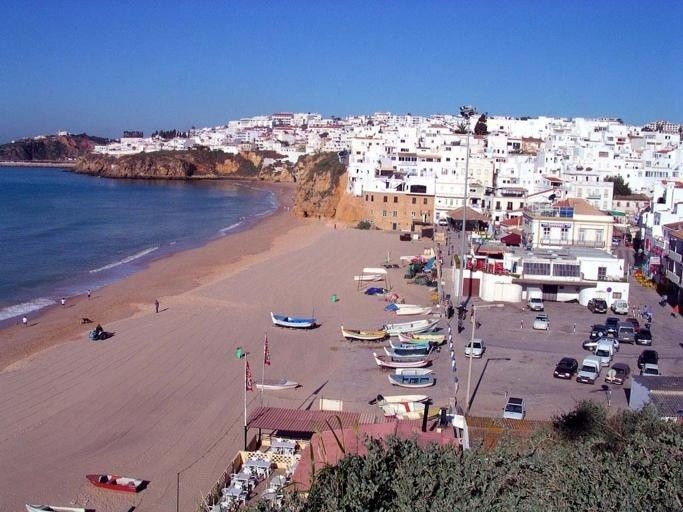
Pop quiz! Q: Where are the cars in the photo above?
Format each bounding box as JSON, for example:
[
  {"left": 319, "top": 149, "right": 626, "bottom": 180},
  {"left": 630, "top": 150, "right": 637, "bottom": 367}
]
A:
[
  {"left": 533, "top": 313, "right": 550, "bottom": 330},
  {"left": 553, "top": 317, "right": 661, "bottom": 385},
  {"left": 464, "top": 338, "right": 484, "bottom": 358}
]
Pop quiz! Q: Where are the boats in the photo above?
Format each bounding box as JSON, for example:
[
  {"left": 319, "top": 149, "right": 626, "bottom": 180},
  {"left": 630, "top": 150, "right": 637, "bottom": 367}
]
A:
[
  {"left": 25, "top": 503, "right": 85, "bottom": 511},
  {"left": 353, "top": 272, "right": 382, "bottom": 281},
  {"left": 368, "top": 302, "right": 446, "bottom": 422},
  {"left": 270, "top": 311, "right": 316, "bottom": 328},
  {"left": 362, "top": 267, "right": 387, "bottom": 275},
  {"left": 85, "top": 474, "right": 144, "bottom": 492},
  {"left": 255, "top": 378, "right": 298, "bottom": 389},
  {"left": 340, "top": 325, "right": 387, "bottom": 340}
]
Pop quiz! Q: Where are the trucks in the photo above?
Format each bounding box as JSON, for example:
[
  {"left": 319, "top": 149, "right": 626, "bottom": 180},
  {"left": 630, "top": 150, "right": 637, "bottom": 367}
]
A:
[{"left": 526, "top": 286, "right": 544, "bottom": 311}]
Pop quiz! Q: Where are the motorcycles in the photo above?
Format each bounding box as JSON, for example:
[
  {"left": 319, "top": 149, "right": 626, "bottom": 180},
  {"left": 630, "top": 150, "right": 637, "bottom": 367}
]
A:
[
  {"left": 88, "top": 329, "right": 108, "bottom": 341},
  {"left": 659, "top": 297, "right": 666, "bottom": 307}
]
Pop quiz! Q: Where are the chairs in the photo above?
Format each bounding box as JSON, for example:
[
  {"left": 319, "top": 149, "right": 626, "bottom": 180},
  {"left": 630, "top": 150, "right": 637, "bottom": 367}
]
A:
[{"left": 211, "top": 437, "right": 300, "bottom": 511}]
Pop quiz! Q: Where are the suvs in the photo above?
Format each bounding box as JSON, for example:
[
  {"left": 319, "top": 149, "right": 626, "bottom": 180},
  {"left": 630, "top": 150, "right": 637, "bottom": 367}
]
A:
[
  {"left": 587, "top": 298, "right": 607, "bottom": 314},
  {"left": 501, "top": 395, "right": 526, "bottom": 420},
  {"left": 610, "top": 300, "right": 629, "bottom": 315}
]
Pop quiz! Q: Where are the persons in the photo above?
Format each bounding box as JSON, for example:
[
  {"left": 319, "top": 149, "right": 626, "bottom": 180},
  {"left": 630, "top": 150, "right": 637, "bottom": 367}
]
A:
[
  {"left": 86, "top": 289, "right": 90, "bottom": 299},
  {"left": 22, "top": 315, "right": 27, "bottom": 328},
  {"left": 81, "top": 318, "right": 94, "bottom": 324},
  {"left": 61, "top": 297, "right": 66, "bottom": 307},
  {"left": 154, "top": 298, "right": 158, "bottom": 312},
  {"left": 94, "top": 323, "right": 103, "bottom": 335}
]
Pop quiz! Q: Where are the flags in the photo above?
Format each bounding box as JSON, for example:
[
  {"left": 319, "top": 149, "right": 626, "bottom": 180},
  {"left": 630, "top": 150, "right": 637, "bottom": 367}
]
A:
[
  {"left": 263, "top": 332, "right": 270, "bottom": 366},
  {"left": 245, "top": 361, "right": 253, "bottom": 392}
]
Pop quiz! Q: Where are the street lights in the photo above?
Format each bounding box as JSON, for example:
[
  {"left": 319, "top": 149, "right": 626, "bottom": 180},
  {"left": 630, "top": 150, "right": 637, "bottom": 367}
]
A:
[
  {"left": 456, "top": 106, "right": 476, "bottom": 307},
  {"left": 465, "top": 303, "right": 504, "bottom": 416}
]
